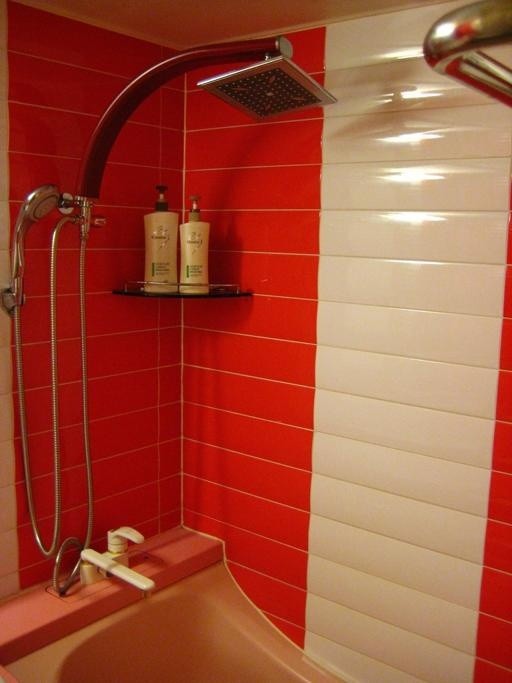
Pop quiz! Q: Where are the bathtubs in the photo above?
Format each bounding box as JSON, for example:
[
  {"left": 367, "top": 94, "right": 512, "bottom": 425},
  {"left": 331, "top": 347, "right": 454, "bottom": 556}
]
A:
[{"left": 3, "top": 560, "right": 353, "bottom": 683}]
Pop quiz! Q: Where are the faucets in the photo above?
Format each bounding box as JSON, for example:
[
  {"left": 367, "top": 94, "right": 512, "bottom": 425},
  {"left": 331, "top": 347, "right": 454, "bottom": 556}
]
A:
[{"left": 80, "top": 548, "right": 155, "bottom": 593}]
